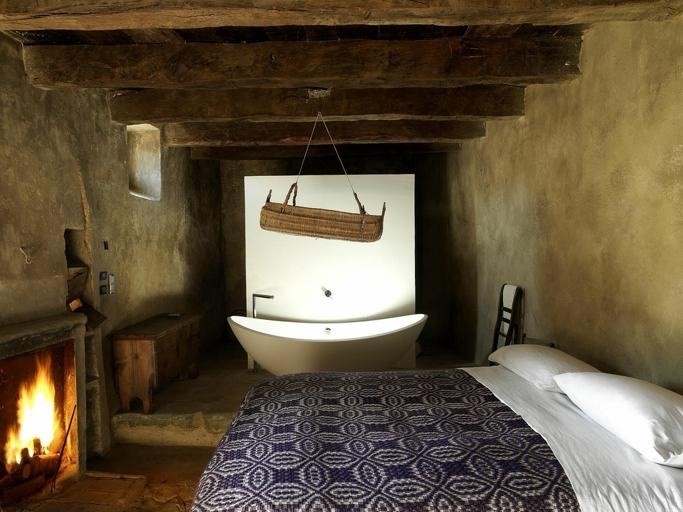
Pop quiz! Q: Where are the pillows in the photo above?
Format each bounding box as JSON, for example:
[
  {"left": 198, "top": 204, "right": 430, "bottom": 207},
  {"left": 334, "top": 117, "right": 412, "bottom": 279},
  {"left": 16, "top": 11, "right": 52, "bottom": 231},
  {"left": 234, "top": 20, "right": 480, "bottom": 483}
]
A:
[
  {"left": 488, "top": 344, "right": 603, "bottom": 394},
  {"left": 553, "top": 371, "right": 683, "bottom": 469}
]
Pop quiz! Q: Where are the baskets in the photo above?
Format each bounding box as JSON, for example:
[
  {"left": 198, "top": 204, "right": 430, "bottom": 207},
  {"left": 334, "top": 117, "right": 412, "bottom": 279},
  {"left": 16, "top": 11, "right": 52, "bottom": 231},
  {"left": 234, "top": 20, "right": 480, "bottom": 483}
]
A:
[{"left": 260, "top": 183, "right": 386, "bottom": 242}]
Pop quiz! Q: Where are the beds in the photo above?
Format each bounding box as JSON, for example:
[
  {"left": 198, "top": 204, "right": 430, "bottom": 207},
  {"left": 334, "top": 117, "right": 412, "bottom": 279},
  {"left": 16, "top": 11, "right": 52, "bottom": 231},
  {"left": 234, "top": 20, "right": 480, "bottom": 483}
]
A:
[{"left": 190, "top": 366, "right": 683, "bottom": 512}]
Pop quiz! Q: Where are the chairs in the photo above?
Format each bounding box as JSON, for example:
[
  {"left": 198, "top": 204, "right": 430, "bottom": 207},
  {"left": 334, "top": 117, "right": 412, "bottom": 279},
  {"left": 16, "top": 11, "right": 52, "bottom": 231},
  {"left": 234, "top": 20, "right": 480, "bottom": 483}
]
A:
[{"left": 490, "top": 283, "right": 525, "bottom": 363}]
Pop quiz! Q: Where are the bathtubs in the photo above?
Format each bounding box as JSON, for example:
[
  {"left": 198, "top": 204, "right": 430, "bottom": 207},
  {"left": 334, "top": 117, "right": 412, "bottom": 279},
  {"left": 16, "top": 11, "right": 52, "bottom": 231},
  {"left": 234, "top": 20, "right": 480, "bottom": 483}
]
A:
[{"left": 228, "top": 311, "right": 427, "bottom": 376}]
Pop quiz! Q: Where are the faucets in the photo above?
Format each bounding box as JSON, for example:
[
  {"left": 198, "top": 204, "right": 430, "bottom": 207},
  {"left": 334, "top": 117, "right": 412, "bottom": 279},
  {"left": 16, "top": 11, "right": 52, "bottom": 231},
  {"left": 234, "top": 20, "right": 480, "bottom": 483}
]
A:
[{"left": 252, "top": 295, "right": 273, "bottom": 317}]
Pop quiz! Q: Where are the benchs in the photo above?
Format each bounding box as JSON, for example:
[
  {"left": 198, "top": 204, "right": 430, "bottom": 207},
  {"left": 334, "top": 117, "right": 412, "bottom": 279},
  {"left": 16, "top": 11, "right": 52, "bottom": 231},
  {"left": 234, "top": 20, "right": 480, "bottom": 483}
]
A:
[{"left": 105, "top": 312, "right": 206, "bottom": 414}]
